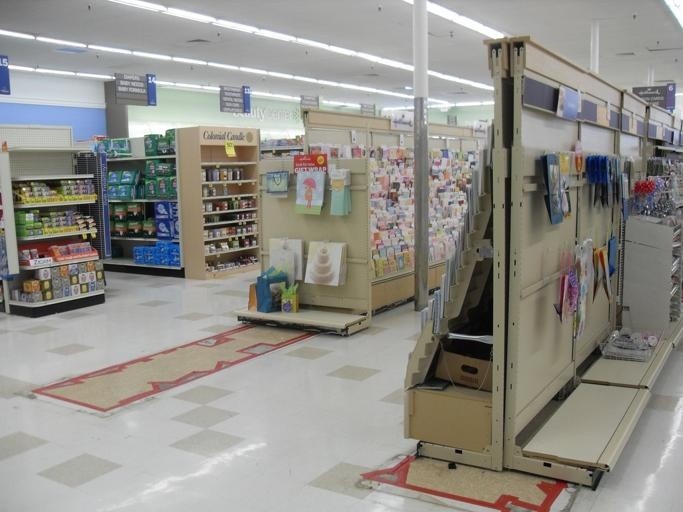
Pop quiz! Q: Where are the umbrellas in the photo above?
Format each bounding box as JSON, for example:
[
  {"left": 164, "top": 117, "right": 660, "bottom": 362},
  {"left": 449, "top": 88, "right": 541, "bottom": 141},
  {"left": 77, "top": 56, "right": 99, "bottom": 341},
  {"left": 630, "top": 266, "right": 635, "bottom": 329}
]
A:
[{"left": 303, "top": 178, "right": 316, "bottom": 189}]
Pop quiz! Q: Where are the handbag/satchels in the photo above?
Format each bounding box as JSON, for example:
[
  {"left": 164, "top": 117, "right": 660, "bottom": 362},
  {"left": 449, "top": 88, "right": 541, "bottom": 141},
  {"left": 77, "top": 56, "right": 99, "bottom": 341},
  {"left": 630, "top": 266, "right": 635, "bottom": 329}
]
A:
[{"left": 248, "top": 170, "right": 351, "bottom": 313}]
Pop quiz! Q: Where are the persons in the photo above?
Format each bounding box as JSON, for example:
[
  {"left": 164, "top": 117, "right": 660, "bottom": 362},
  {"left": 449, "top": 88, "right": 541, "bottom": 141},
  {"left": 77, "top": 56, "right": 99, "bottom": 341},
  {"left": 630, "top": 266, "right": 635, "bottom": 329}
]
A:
[{"left": 305, "top": 186, "right": 313, "bottom": 209}]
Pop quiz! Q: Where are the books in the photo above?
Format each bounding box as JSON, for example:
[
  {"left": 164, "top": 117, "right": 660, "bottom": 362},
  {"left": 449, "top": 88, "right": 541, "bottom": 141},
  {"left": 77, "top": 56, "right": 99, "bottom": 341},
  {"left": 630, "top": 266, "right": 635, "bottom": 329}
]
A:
[{"left": 420, "top": 122, "right": 493, "bottom": 334}]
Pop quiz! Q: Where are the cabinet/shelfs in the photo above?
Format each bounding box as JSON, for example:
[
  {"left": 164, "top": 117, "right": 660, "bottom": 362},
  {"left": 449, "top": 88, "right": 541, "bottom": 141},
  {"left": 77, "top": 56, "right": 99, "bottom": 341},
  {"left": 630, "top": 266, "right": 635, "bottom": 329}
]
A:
[
  {"left": 105, "top": 129, "right": 184, "bottom": 270},
  {"left": 0, "top": 146, "right": 106, "bottom": 318},
  {"left": 199, "top": 127, "right": 261, "bottom": 279}
]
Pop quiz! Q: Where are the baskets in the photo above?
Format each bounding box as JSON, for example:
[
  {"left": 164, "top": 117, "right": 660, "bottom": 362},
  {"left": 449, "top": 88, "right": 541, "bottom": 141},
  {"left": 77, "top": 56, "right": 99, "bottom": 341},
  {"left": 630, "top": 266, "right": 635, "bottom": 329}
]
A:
[{"left": 597, "top": 325, "right": 664, "bottom": 361}]
